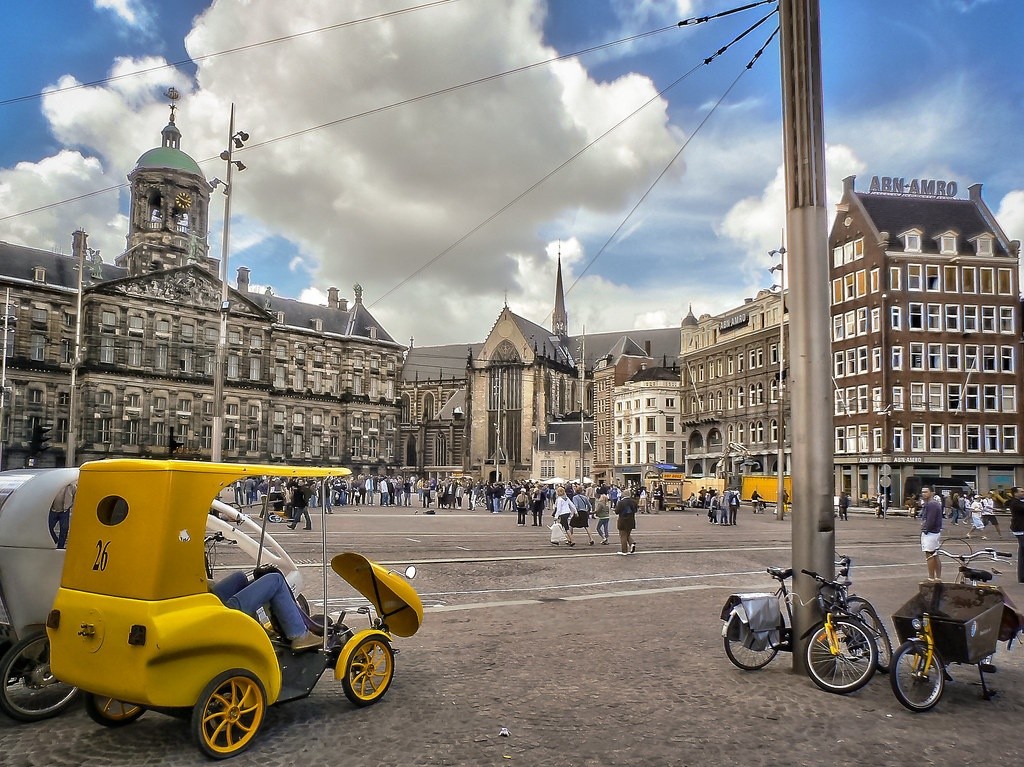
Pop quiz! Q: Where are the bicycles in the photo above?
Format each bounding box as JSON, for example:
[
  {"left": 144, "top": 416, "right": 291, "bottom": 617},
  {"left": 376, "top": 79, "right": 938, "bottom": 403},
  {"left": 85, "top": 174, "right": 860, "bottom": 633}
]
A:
[
  {"left": 718, "top": 552, "right": 895, "bottom": 694},
  {"left": 885, "top": 531, "right": 1014, "bottom": 711}
]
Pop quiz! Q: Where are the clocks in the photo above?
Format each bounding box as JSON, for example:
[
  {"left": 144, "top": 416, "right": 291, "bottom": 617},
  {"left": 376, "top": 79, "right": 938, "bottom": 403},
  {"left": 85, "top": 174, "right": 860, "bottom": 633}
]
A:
[{"left": 176, "top": 191, "right": 192, "bottom": 208}]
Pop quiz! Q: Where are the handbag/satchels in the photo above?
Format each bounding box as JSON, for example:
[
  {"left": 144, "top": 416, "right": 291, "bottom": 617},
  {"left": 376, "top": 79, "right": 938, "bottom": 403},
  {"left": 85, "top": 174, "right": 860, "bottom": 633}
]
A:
[
  {"left": 335, "top": 493, "right": 339, "bottom": 500},
  {"left": 519, "top": 502, "right": 524, "bottom": 507},
  {"left": 720, "top": 591, "right": 781, "bottom": 652},
  {"left": 355, "top": 492, "right": 360, "bottom": 497},
  {"left": 547, "top": 521, "right": 567, "bottom": 543}
]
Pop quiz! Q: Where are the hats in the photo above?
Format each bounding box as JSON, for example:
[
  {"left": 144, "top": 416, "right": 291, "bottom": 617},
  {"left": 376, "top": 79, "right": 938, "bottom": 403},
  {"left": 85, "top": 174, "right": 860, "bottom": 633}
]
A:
[
  {"left": 988, "top": 491, "right": 995, "bottom": 495},
  {"left": 298, "top": 480, "right": 304, "bottom": 486},
  {"left": 974, "top": 495, "right": 981, "bottom": 499},
  {"left": 520, "top": 488, "right": 526, "bottom": 493}
]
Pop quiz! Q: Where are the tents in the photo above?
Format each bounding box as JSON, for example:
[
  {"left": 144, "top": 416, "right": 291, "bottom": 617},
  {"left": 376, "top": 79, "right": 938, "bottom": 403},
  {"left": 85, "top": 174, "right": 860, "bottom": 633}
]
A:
[{"left": 525, "top": 477, "right": 596, "bottom": 489}]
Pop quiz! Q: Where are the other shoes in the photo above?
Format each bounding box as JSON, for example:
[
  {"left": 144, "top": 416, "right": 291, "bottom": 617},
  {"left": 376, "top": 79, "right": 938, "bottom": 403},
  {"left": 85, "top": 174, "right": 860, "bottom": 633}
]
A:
[
  {"left": 303, "top": 527, "right": 310, "bottom": 530},
  {"left": 589, "top": 540, "right": 594, "bottom": 546},
  {"left": 630, "top": 544, "right": 636, "bottom": 553},
  {"left": 600, "top": 539, "right": 609, "bottom": 545},
  {"left": 709, "top": 520, "right": 738, "bottom": 526},
  {"left": 617, "top": 551, "right": 627, "bottom": 555},
  {"left": 565, "top": 540, "right": 571, "bottom": 544},
  {"left": 570, "top": 542, "right": 575, "bottom": 546},
  {"left": 286, "top": 524, "right": 294, "bottom": 530},
  {"left": 951, "top": 521, "right": 1004, "bottom": 540},
  {"left": 368, "top": 502, "right": 517, "bottom": 513}
]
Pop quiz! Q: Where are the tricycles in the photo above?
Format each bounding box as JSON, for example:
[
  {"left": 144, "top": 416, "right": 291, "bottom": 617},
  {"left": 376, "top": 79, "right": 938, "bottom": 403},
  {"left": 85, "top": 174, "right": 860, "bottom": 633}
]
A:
[
  {"left": 0, "top": 467, "right": 303, "bottom": 725},
  {"left": 46, "top": 455, "right": 425, "bottom": 760}
]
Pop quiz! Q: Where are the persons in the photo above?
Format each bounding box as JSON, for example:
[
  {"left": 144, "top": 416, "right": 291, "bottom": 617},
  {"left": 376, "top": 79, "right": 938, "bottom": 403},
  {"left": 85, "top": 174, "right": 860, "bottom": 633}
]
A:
[
  {"left": 839, "top": 491, "right": 848, "bottom": 521},
  {"left": 531, "top": 488, "right": 546, "bottom": 526},
  {"left": 752, "top": 491, "right": 762, "bottom": 514},
  {"left": 264, "top": 286, "right": 274, "bottom": 308},
  {"left": 287, "top": 479, "right": 312, "bottom": 530},
  {"left": 93, "top": 250, "right": 102, "bottom": 277},
  {"left": 783, "top": 490, "right": 788, "bottom": 504},
  {"left": 231, "top": 473, "right": 413, "bottom": 521},
  {"left": 515, "top": 488, "right": 529, "bottom": 527},
  {"left": 615, "top": 490, "right": 638, "bottom": 556},
  {"left": 709, "top": 487, "right": 740, "bottom": 526},
  {"left": 524, "top": 480, "right": 648, "bottom": 519},
  {"left": 1010, "top": 487, "right": 1024, "bottom": 584},
  {"left": 410, "top": 473, "right": 526, "bottom": 514},
  {"left": 876, "top": 488, "right": 1016, "bottom": 540},
  {"left": 49, "top": 482, "right": 77, "bottom": 549},
  {"left": 920, "top": 484, "right": 943, "bottom": 582},
  {"left": 550, "top": 487, "right": 610, "bottom": 547},
  {"left": 210, "top": 570, "right": 329, "bottom": 649},
  {"left": 687, "top": 487, "right": 708, "bottom": 509}
]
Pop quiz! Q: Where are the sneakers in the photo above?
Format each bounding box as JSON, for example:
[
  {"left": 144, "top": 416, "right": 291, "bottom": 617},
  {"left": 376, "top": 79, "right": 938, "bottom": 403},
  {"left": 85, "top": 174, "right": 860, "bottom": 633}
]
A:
[{"left": 290, "top": 630, "right": 325, "bottom": 649}]
